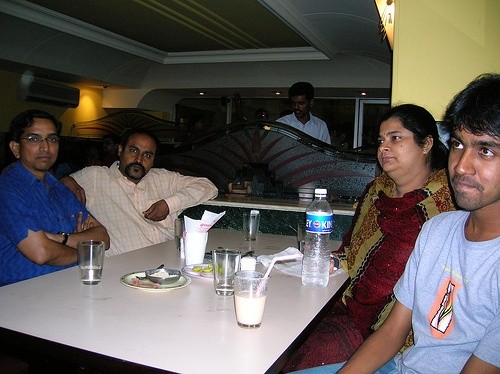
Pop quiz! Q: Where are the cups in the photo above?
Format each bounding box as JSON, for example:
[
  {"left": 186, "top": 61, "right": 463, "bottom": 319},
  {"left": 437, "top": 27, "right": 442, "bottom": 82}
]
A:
[
  {"left": 173, "top": 218, "right": 185, "bottom": 250},
  {"left": 297, "top": 223, "right": 308, "bottom": 255},
  {"left": 77, "top": 240, "right": 104, "bottom": 285},
  {"left": 212, "top": 248, "right": 241, "bottom": 296},
  {"left": 242, "top": 212, "right": 260, "bottom": 241},
  {"left": 183, "top": 231, "right": 208, "bottom": 264},
  {"left": 234, "top": 270, "right": 269, "bottom": 329}
]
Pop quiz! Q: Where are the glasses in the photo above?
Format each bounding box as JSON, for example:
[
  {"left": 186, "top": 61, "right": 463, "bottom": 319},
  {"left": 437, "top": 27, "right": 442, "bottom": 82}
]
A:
[
  {"left": 17, "top": 135, "right": 60, "bottom": 143},
  {"left": 103, "top": 142, "right": 115, "bottom": 146}
]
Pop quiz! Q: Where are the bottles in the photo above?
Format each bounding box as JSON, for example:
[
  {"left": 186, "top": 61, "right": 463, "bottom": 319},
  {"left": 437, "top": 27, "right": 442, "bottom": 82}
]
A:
[
  {"left": 240, "top": 251, "right": 257, "bottom": 272},
  {"left": 301, "top": 189, "right": 334, "bottom": 288},
  {"left": 71, "top": 121, "right": 78, "bottom": 136}
]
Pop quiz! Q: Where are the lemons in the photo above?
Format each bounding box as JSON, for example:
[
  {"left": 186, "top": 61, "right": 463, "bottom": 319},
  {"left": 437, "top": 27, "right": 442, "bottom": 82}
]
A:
[{"left": 193, "top": 264, "right": 213, "bottom": 272}]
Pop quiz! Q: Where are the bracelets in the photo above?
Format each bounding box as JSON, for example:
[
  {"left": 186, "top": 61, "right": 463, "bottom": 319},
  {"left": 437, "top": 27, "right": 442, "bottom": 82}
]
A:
[{"left": 331, "top": 256, "right": 338, "bottom": 271}]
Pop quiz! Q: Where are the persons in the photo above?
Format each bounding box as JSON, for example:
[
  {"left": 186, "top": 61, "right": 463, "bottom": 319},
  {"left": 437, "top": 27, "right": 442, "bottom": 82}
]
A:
[
  {"left": 150, "top": 145, "right": 184, "bottom": 174},
  {"left": 275, "top": 82, "right": 331, "bottom": 145},
  {"left": 285, "top": 71, "right": 500, "bottom": 374},
  {"left": 280, "top": 104, "right": 458, "bottom": 374},
  {"left": 0, "top": 109, "right": 110, "bottom": 288},
  {"left": 59, "top": 127, "right": 219, "bottom": 259},
  {"left": 71, "top": 132, "right": 121, "bottom": 171}
]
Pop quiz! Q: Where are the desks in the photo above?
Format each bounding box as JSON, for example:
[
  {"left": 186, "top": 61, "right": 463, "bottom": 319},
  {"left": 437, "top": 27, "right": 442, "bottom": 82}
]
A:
[
  {"left": 0, "top": 229, "right": 349, "bottom": 374},
  {"left": 205, "top": 194, "right": 358, "bottom": 216}
]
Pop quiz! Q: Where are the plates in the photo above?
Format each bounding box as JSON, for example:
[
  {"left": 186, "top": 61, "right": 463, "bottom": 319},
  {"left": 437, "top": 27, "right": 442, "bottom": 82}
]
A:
[
  {"left": 145, "top": 268, "right": 181, "bottom": 285},
  {"left": 182, "top": 263, "right": 216, "bottom": 281},
  {"left": 120, "top": 270, "right": 191, "bottom": 292}
]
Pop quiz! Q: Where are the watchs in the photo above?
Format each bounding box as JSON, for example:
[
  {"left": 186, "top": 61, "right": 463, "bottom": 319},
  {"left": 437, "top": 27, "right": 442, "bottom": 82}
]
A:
[{"left": 57, "top": 231, "right": 70, "bottom": 246}]
